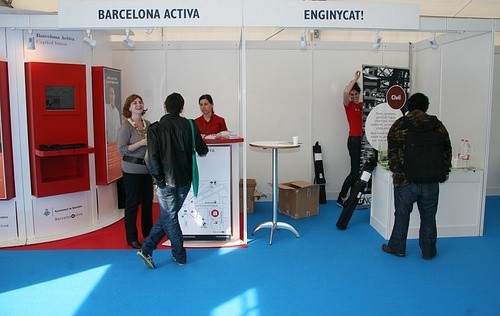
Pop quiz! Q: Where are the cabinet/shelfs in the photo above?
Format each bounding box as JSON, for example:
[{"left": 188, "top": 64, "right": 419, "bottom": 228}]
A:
[{"left": 370, "top": 161, "right": 484, "bottom": 240}]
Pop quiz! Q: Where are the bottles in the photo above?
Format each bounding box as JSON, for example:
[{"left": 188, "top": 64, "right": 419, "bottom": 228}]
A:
[{"left": 458, "top": 138, "right": 471, "bottom": 167}]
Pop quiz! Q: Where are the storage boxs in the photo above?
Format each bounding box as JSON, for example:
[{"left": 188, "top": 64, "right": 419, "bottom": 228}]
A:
[
  {"left": 240, "top": 179, "right": 257, "bottom": 213},
  {"left": 278, "top": 181, "right": 320, "bottom": 220}
]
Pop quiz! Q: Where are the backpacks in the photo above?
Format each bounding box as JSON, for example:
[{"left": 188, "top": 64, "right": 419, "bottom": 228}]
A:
[{"left": 402, "top": 115, "right": 445, "bottom": 184}]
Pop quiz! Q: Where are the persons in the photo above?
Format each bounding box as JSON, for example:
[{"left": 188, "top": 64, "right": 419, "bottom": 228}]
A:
[
  {"left": 106, "top": 84, "right": 121, "bottom": 144},
  {"left": 117, "top": 94, "right": 153, "bottom": 249},
  {"left": 194, "top": 93, "right": 227, "bottom": 136},
  {"left": 336, "top": 70, "right": 363, "bottom": 208},
  {"left": 382, "top": 92, "right": 452, "bottom": 259},
  {"left": 137, "top": 95, "right": 209, "bottom": 270}
]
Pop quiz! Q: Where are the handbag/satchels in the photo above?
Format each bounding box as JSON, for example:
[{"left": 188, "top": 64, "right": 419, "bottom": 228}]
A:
[{"left": 189, "top": 119, "right": 199, "bottom": 197}]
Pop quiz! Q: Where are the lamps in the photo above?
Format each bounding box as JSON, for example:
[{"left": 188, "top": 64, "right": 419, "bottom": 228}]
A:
[
  {"left": 27, "top": 29, "right": 35, "bottom": 50},
  {"left": 429, "top": 37, "right": 440, "bottom": 49},
  {"left": 123, "top": 28, "right": 135, "bottom": 49},
  {"left": 373, "top": 34, "right": 382, "bottom": 50},
  {"left": 298, "top": 33, "right": 308, "bottom": 48},
  {"left": 83, "top": 29, "right": 96, "bottom": 49}
]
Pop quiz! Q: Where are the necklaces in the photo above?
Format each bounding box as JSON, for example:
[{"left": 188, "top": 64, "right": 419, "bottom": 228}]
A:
[{"left": 130, "top": 118, "right": 145, "bottom": 137}]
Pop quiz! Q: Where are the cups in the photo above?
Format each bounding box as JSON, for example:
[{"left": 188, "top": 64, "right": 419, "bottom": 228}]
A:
[
  {"left": 293, "top": 136, "right": 298, "bottom": 145},
  {"left": 228, "top": 130, "right": 235, "bottom": 138}
]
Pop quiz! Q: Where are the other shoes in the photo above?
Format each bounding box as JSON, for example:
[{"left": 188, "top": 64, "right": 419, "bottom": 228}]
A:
[
  {"left": 422, "top": 252, "right": 436, "bottom": 259},
  {"left": 137, "top": 248, "right": 155, "bottom": 269},
  {"left": 172, "top": 256, "right": 185, "bottom": 265},
  {"left": 336, "top": 197, "right": 346, "bottom": 207},
  {"left": 382, "top": 244, "right": 405, "bottom": 256}
]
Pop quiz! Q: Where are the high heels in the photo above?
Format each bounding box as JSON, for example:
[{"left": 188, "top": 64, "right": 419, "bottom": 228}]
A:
[{"left": 128, "top": 241, "right": 142, "bottom": 249}]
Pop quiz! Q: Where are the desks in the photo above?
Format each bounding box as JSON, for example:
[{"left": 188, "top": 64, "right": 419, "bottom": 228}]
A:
[{"left": 249, "top": 140, "right": 301, "bottom": 246}]
